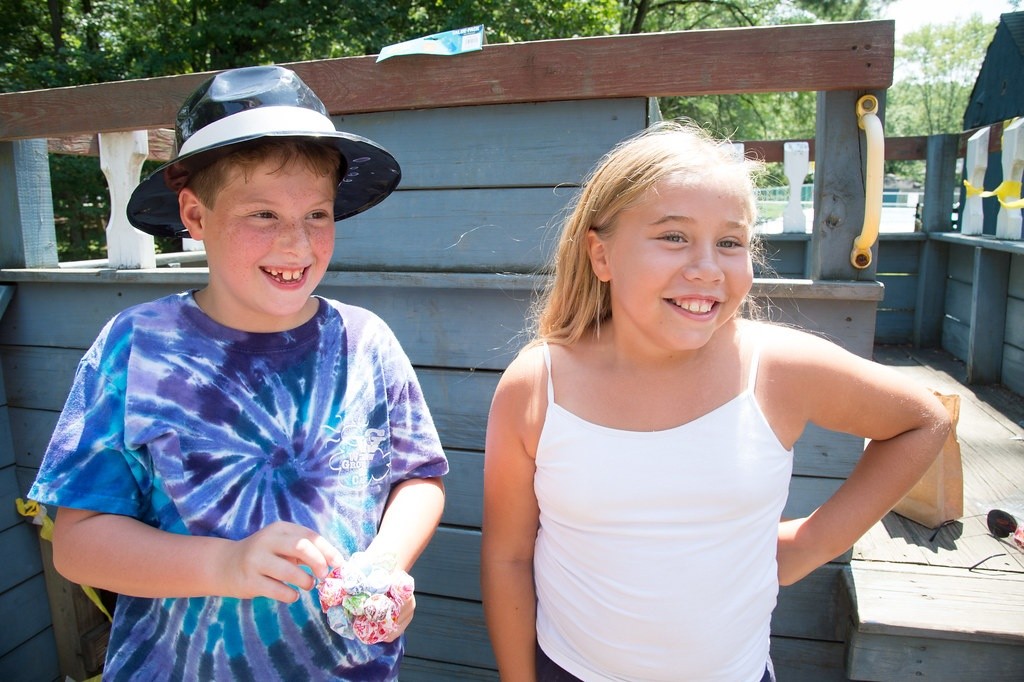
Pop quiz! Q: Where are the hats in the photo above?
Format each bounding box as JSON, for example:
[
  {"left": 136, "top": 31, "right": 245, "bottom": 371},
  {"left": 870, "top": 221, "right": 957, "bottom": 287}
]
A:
[{"left": 126, "top": 66, "right": 401, "bottom": 238}]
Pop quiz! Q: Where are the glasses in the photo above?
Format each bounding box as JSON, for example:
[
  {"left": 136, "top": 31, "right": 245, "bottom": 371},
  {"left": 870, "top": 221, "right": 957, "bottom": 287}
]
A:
[{"left": 927, "top": 507, "right": 1024, "bottom": 570}]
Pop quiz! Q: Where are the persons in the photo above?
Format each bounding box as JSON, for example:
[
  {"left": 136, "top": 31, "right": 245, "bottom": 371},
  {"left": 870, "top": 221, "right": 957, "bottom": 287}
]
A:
[
  {"left": 480, "top": 125, "right": 956, "bottom": 682},
  {"left": 27, "top": 64, "right": 450, "bottom": 680}
]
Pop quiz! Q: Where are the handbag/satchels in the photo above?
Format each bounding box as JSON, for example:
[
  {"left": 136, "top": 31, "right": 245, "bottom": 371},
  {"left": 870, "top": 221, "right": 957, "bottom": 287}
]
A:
[{"left": 875, "top": 379, "right": 964, "bottom": 529}]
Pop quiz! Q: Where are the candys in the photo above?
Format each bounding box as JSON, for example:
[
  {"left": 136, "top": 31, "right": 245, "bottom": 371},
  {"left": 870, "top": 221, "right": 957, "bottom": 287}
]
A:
[{"left": 316, "top": 561, "right": 414, "bottom": 644}]
